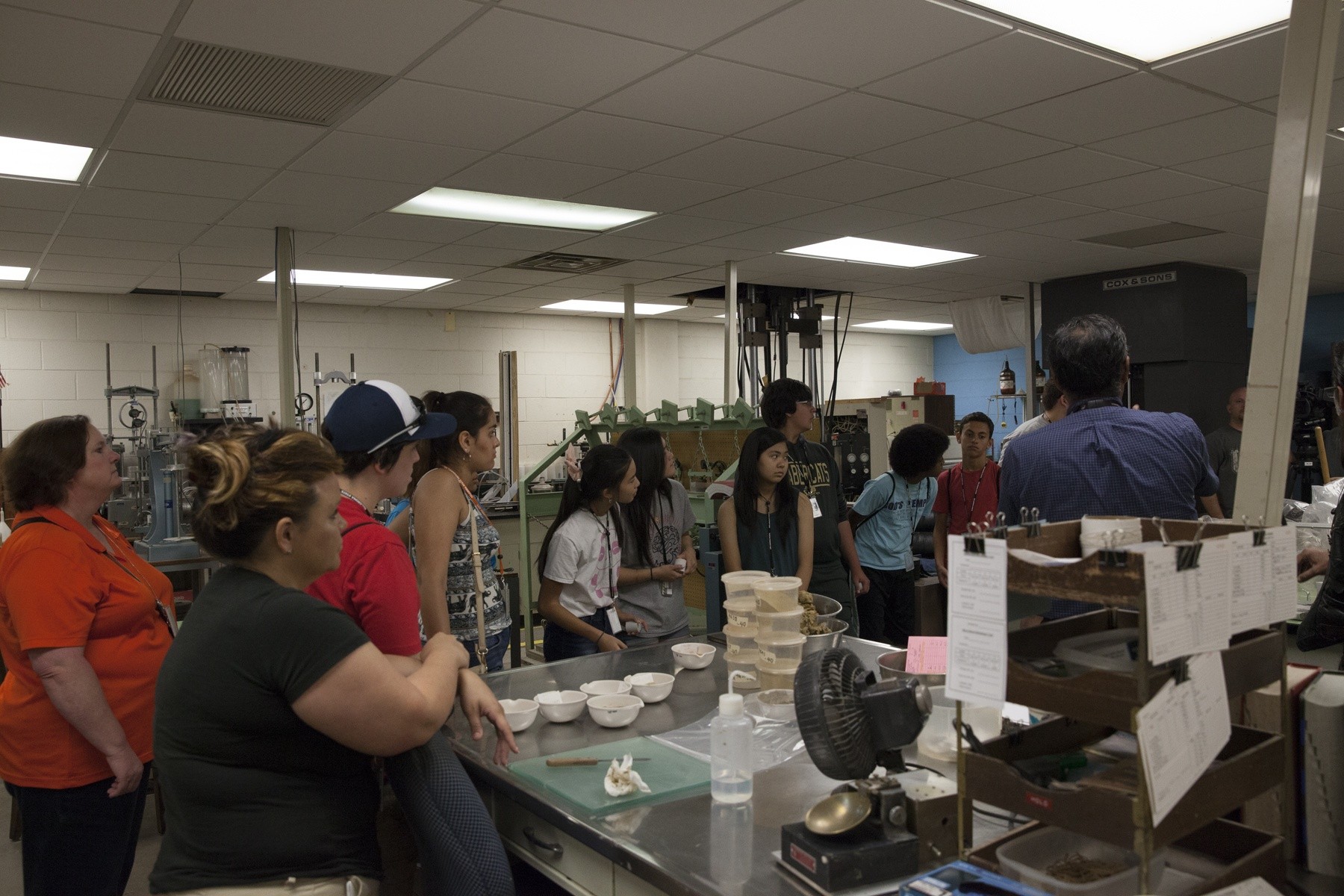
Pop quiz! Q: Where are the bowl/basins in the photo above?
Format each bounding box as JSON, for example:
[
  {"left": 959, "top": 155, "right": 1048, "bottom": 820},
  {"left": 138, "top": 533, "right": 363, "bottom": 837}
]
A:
[
  {"left": 799, "top": 594, "right": 843, "bottom": 617},
  {"left": 580, "top": 680, "right": 632, "bottom": 702},
  {"left": 757, "top": 658, "right": 803, "bottom": 688},
  {"left": 721, "top": 570, "right": 770, "bottom": 605},
  {"left": 722, "top": 624, "right": 757, "bottom": 656},
  {"left": 876, "top": 649, "right": 946, "bottom": 687},
  {"left": 756, "top": 689, "right": 795, "bottom": 719},
  {"left": 751, "top": 576, "right": 802, "bottom": 612},
  {"left": 1052, "top": 628, "right": 1178, "bottom": 686},
  {"left": 798, "top": 618, "right": 849, "bottom": 660},
  {"left": 533, "top": 690, "right": 589, "bottom": 723},
  {"left": 917, "top": 686, "right": 1003, "bottom": 761},
  {"left": 722, "top": 649, "right": 762, "bottom": 689},
  {"left": 754, "top": 605, "right": 804, "bottom": 634},
  {"left": 671, "top": 643, "right": 717, "bottom": 669},
  {"left": 624, "top": 673, "right": 675, "bottom": 704},
  {"left": 995, "top": 826, "right": 1168, "bottom": 896},
  {"left": 586, "top": 694, "right": 645, "bottom": 727},
  {"left": 723, "top": 597, "right": 758, "bottom": 627},
  {"left": 755, "top": 631, "right": 807, "bottom": 670},
  {"left": 496, "top": 699, "right": 539, "bottom": 732}
]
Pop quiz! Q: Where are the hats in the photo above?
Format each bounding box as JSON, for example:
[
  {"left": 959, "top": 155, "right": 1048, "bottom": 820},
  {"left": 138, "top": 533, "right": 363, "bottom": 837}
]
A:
[{"left": 321, "top": 379, "right": 458, "bottom": 455}]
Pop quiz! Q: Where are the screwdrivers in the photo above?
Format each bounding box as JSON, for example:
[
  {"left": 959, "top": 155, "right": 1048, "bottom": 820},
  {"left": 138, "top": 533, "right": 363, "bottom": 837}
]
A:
[{"left": 546, "top": 757, "right": 651, "bottom": 766}]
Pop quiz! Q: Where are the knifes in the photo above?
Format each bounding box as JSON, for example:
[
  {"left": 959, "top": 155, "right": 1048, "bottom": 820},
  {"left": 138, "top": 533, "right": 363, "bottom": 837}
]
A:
[{"left": 546, "top": 758, "right": 652, "bottom": 767}]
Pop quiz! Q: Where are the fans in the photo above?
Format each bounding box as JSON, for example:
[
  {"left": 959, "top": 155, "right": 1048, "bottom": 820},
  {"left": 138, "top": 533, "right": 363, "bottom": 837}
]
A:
[{"left": 793, "top": 646, "right": 932, "bottom": 779}]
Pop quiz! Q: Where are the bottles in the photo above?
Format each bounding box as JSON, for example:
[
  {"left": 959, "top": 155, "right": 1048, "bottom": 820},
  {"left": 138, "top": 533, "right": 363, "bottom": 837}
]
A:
[
  {"left": 1000, "top": 361, "right": 1015, "bottom": 394},
  {"left": 710, "top": 694, "right": 753, "bottom": 803},
  {"left": 1035, "top": 360, "right": 1046, "bottom": 393}
]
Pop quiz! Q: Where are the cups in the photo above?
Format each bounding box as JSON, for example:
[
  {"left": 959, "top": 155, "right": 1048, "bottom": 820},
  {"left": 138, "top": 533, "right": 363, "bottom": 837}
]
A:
[
  {"left": 674, "top": 558, "right": 686, "bottom": 573},
  {"left": 626, "top": 621, "right": 641, "bottom": 633}
]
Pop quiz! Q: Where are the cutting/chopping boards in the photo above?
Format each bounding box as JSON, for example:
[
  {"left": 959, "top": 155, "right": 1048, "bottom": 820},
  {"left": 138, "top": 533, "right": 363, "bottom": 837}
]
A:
[{"left": 508, "top": 735, "right": 710, "bottom": 813}]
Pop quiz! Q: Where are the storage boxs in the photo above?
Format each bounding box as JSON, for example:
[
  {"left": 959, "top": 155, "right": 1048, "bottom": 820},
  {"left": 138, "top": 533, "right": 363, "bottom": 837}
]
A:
[{"left": 995, "top": 826, "right": 1168, "bottom": 896}]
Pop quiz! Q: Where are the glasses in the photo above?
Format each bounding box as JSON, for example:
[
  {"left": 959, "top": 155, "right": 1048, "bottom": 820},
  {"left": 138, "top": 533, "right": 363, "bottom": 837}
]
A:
[{"left": 367, "top": 395, "right": 428, "bottom": 454}]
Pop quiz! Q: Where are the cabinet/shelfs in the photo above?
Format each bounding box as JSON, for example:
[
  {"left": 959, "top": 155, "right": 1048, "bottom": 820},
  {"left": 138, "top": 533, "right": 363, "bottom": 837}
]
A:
[{"left": 953, "top": 515, "right": 1295, "bottom": 896}]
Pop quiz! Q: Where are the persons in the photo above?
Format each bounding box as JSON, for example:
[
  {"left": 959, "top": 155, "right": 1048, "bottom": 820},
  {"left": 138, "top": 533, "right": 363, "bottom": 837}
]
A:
[
  {"left": 532, "top": 442, "right": 649, "bottom": 666},
  {"left": 0, "top": 415, "right": 179, "bottom": 896},
  {"left": 848, "top": 424, "right": 951, "bottom": 649},
  {"left": 996, "top": 315, "right": 1344, "bottom": 583},
  {"left": 717, "top": 427, "right": 815, "bottom": 593},
  {"left": 760, "top": 377, "right": 871, "bottom": 638},
  {"left": 301, "top": 380, "right": 512, "bottom": 674},
  {"left": 932, "top": 412, "right": 1003, "bottom": 589},
  {"left": 152, "top": 423, "right": 519, "bottom": 896},
  {"left": 616, "top": 426, "right": 698, "bottom": 651}
]
